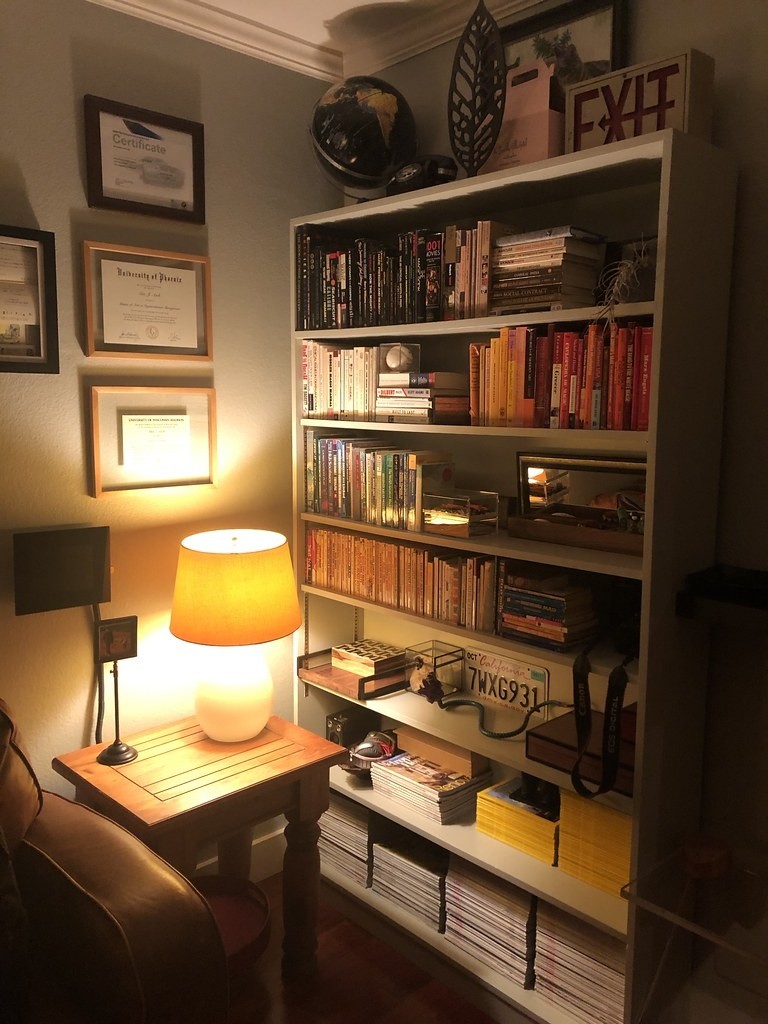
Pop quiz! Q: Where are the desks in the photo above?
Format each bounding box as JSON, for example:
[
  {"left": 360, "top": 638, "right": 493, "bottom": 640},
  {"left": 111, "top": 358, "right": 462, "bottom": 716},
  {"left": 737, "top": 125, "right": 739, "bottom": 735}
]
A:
[{"left": 51, "top": 715, "right": 350, "bottom": 1004}]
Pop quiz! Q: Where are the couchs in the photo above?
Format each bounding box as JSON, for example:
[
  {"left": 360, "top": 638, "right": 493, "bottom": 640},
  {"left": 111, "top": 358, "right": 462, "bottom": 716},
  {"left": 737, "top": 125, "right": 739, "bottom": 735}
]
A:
[{"left": 1, "top": 698, "right": 230, "bottom": 1024}]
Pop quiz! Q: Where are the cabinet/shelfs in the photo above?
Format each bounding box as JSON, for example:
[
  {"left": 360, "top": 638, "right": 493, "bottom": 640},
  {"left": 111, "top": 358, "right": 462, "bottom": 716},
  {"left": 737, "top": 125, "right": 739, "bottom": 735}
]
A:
[{"left": 288, "top": 126, "right": 736, "bottom": 1024}]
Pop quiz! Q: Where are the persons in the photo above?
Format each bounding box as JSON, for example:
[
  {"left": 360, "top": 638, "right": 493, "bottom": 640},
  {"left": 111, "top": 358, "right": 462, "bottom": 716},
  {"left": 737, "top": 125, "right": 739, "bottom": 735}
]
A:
[{"left": 482, "top": 264, "right": 487, "bottom": 278}]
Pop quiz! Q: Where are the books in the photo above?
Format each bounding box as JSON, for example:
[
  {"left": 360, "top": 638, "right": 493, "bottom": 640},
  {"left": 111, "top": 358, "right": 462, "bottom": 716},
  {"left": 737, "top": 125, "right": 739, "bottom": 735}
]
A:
[{"left": 296, "top": 225, "right": 652, "bottom": 1024}]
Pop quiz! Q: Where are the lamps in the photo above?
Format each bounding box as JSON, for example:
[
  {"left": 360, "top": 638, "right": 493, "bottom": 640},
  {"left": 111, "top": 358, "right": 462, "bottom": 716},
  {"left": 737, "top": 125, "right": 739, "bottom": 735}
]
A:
[{"left": 168, "top": 528, "right": 301, "bottom": 744}]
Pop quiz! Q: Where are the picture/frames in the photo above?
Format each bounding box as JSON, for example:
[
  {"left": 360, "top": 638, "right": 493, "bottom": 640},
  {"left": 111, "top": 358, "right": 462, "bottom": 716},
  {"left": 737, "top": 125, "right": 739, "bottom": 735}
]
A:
[
  {"left": 85, "top": 93, "right": 206, "bottom": 225},
  {"left": 480, "top": 0, "right": 628, "bottom": 110},
  {"left": 515, "top": 451, "right": 646, "bottom": 517},
  {"left": 0, "top": 224, "right": 60, "bottom": 376},
  {"left": 89, "top": 385, "right": 217, "bottom": 498},
  {"left": 82, "top": 240, "right": 212, "bottom": 361},
  {"left": 95, "top": 615, "right": 137, "bottom": 665}
]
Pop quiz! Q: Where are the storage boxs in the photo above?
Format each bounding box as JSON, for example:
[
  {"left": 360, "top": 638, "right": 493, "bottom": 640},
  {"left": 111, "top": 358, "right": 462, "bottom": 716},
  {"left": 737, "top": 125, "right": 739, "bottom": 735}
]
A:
[
  {"left": 475, "top": 60, "right": 568, "bottom": 175},
  {"left": 508, "top": 502, "right": 644, "bottom": 556},
  {"left": 380, "top": 343, "right": 421, "bottom": 373},
  {"left": 405, "top": 640, "right": 466, "bottom": 698},
  {"left": 564, "top": 48, "right": 715, "bottom": 155},
  {"left": 422, "top": 489, "right": 499, "bottom": 540},
  {"left": 526, "top": 708, "right": 633, "bottom": 798},
  {"left": 393, "top": 726, "right": 490, "bottom": 779}
]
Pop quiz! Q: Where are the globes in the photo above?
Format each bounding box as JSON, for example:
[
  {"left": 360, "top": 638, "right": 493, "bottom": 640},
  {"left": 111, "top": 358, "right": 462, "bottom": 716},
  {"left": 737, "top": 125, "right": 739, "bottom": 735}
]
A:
[{"left": 307, "top": 75, "right": 418, "bottom": 208}]
